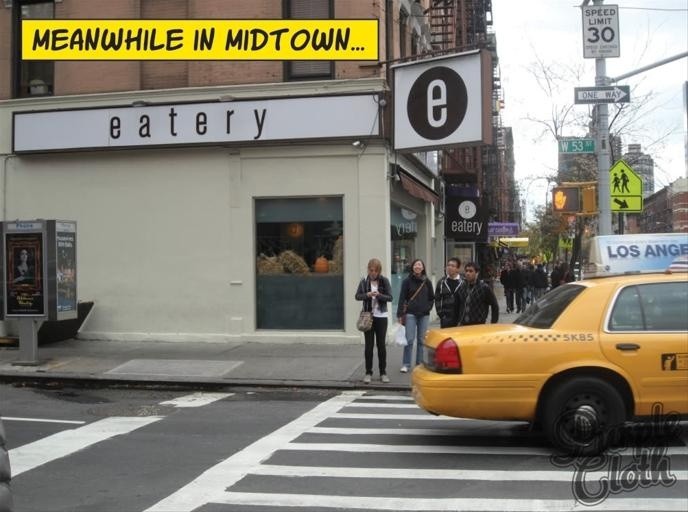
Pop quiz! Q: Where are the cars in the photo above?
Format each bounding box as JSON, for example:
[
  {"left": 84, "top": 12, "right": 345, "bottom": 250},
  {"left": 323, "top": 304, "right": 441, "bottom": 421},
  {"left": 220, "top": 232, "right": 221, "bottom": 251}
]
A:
[
  {"left": 406, "top": 231, "right": 687, "bottom": 460},
  {"left": 572, "top": 260, "right": 579, "bottom": 277}
]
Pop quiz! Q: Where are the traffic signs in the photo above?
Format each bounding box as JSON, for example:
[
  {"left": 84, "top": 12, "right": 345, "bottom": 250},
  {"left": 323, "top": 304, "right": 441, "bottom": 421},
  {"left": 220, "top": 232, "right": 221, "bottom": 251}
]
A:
[
  {"left": 557, "top": 139, "right": 594, "bottom": 155},
  {"left": 574, "top": 85, "right": 631, "bottom": 104},
  {"left": 581, "top": 4, "right": 621, "bottom": 59}
]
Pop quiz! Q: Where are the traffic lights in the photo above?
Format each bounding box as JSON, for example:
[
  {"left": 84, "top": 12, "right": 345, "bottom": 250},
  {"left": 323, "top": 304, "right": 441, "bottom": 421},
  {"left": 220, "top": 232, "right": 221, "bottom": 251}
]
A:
[{"left": 551, "top": 184, "right": 596, "bottom": 215}]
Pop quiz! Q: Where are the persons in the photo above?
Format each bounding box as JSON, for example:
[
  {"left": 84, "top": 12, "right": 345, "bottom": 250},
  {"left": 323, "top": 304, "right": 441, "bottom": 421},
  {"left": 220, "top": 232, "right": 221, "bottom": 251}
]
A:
[
  {"left": 14, "top": 247, "right": 34, "bottom": 284},
  {"left": 435, "top": 257, "right": 466, "bottom": 329},
  {"left": 397, "top": 258, "right": 435, "bottom": 373},
  {"left": 456, "top": 262, "right": 499, "bottom": 327},
  {"left": 355, "top": 258, "right": 393, "bottom": 383},
  {"left": 500, "top": 259, "right": 575, "bottom": 314}
]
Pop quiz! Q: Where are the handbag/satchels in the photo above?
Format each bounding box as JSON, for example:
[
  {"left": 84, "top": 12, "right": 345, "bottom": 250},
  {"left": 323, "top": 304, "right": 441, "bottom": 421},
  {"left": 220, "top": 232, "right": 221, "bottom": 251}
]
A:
[
  {"left": 399, "top": 303, "right": 409, "bottom": 326},
  {"left": 355, "top": 311, "right": 374, "bottom": 332}
]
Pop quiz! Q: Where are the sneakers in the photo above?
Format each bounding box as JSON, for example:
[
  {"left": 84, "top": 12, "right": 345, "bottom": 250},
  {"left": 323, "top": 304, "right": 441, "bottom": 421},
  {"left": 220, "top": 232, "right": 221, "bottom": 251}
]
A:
[
  {"left": 363, "top": 374, "right": 372, "bottom": 383},
  {"left": 379, "top": 375, "right": 390, "bottom": 383},
  {"left": 400, "top": 366, "right": 410, "bottom": 372}
]
